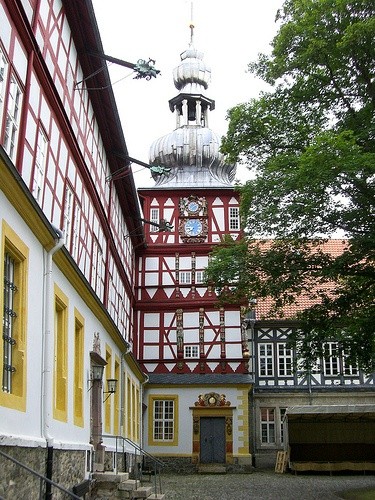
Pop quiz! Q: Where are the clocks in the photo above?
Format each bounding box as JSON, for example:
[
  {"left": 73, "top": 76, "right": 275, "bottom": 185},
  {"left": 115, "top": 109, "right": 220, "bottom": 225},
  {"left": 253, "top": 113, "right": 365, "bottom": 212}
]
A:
[
  {"left": 184, "top": 219, "right": 202, "bottom": 237},
  {"left": 186, "top": 200, "right": 200, "bottom": 213}
]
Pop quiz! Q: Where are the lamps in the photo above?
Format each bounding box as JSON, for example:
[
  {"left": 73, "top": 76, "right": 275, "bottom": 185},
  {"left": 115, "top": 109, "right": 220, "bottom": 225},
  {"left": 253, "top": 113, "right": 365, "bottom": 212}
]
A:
[
  {"left": 85, "top": 364, "right": 104, "bottom": 392},
  {"left": 102, "top": 378, "right": 118, "bottom": 403}
]
